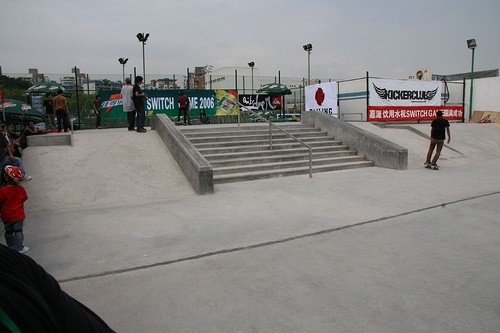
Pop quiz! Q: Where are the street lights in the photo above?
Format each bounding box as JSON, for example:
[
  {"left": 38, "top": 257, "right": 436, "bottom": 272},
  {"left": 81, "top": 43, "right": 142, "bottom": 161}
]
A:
[
  {"left": 137, "top": 31, "right": 150, "bottom": 91},
  {"left": 118, "top": 57, "right": 129, "bottom": 84},
  {"left": 303, "top": 44, "right": 312, "bottom": 85},
  {"left": 466, "top": 38, "right": 477, "bottom": 120},
  {"left": 247, "top": 62, "right": 255, "bottom": 94}
]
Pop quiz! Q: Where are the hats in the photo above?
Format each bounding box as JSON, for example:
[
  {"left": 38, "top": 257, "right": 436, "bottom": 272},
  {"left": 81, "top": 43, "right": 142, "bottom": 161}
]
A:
[{"left": 4, "top": 165, "right": 25, "bottom": 183}]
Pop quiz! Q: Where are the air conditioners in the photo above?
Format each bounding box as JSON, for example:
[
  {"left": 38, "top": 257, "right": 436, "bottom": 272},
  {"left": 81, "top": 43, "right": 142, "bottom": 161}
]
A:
[{"left": 415, "top": 68, "right": 432, "bottom": 80}]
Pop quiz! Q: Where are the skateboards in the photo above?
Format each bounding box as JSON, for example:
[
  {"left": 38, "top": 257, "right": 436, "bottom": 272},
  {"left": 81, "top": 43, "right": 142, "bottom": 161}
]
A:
[{"left": 424, "top": 162, "right": 441, "bottom": 170}]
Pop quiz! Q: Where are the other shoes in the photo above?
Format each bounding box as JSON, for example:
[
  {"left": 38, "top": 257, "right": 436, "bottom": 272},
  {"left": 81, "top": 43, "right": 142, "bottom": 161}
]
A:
[
  {"left": 24, "top": 174, "right": 32, "bottom": 181},
  {"left": 128, "top": 128, "right": 137, "bottom": 131},
  {"left": 426, "top": 161, "right": 431, "bottom": 164},
  {"left": 137, "top": 127, "right": 146, "bottom": 132},
  {"left": 432, "top": 164, "right": 437, "bottom": 166},
  {"left": 19, "top": 246, "right": 30, "bottom": 253}
]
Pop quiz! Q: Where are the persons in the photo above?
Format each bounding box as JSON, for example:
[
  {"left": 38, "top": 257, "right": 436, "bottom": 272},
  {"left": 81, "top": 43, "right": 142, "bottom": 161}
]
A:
[
  {"left": 132, "top": 75, "right": 148, "bottom": 133},
  {"left": 53, "top": 88, "right": 71, "bottom": 134},
  {"left": 119, "top": 77, "right": 137, "bottom": 131},
  {"left": 0, "top": 120, "right": 41, "bottom": 255},
  {"left": 425, "top": 109, "right": 451, "bottom": 167},
  {"left": 177, "top": 92, "right": 193, "bottom": 125},
  {"left": 198, "top": 108, "right": 211, "bottom": 125},
  {"left": 41, "top": 92, "right": 56, "bottom": 133},
  {"left": 0, "top": 243, "right": 115, "bottom": 333},
  {"left": 91, "top": 94, "right": 105, "bottom": 129}
]
